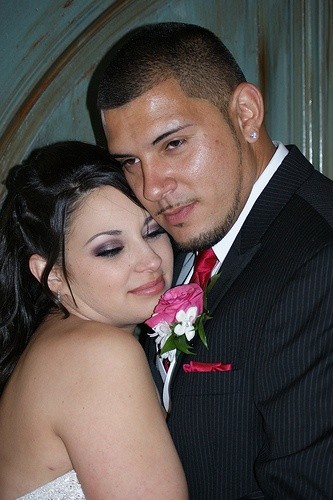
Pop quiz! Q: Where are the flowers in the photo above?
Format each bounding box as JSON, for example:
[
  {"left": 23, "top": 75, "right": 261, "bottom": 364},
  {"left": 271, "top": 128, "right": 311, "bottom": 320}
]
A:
[{"left": 145, "top": 271, "right": 223, "bottom": 362}]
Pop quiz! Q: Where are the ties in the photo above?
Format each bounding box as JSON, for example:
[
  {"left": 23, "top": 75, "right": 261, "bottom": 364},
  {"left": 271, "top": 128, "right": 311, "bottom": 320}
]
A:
[{"left": 188, "top": 248, "right": 216, "bottom": 290}]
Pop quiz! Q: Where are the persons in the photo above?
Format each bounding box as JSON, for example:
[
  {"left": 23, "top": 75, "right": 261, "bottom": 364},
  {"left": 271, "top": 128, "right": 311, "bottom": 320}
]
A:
[
  {"left": 1, "top": 139, "right": 192, "bottom": 500},
  {"left": 85, "top": 20, "right": 333, "bottom": 499}
]
